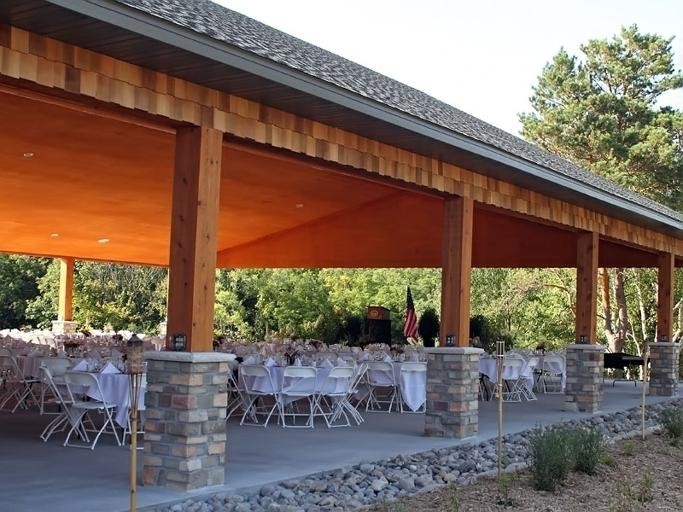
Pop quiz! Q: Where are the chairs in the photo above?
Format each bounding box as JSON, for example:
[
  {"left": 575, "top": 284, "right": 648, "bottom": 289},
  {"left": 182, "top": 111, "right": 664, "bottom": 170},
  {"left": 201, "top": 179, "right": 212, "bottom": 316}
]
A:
[
  {"left": 227, "top": 344, "right": 427, "bottom": 429},
  {"left": 1, "top": 340, "right": 147, "bottom": 451},
  {"left": 478, "top": 347, "right": 566, "bottom": 404}
]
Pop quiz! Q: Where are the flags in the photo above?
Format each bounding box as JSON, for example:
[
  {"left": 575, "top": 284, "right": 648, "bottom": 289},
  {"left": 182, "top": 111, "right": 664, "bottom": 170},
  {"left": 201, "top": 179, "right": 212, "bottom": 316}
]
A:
[{"left": 404, "top": 287, "right": 419, "bottom": 341}]
